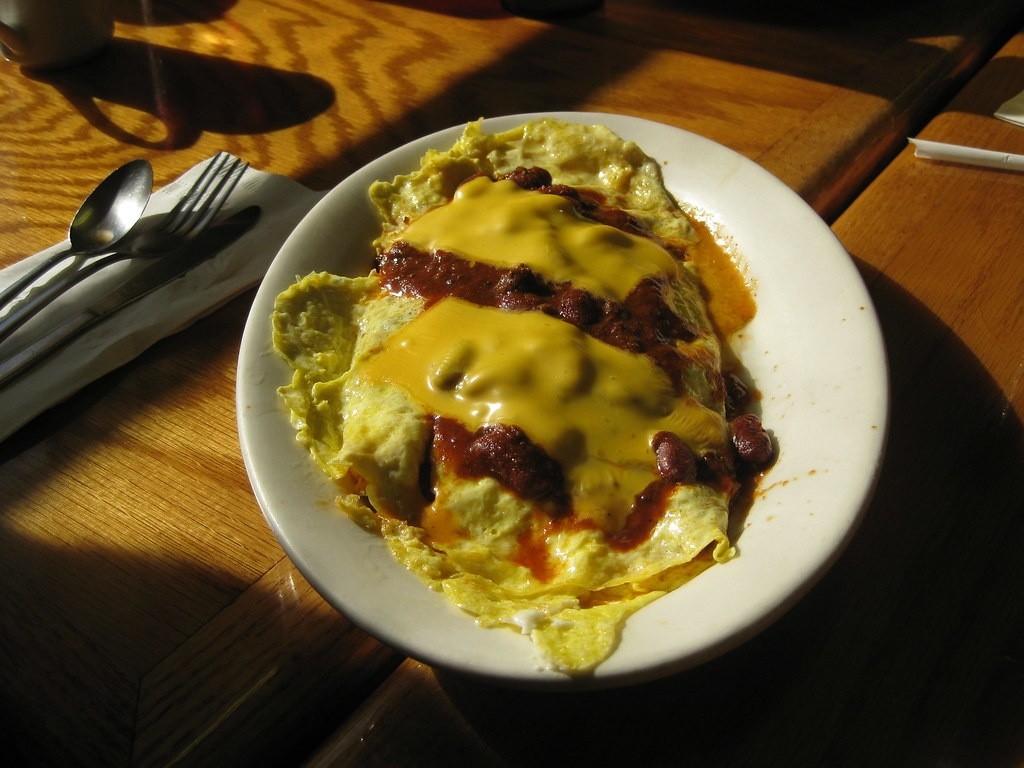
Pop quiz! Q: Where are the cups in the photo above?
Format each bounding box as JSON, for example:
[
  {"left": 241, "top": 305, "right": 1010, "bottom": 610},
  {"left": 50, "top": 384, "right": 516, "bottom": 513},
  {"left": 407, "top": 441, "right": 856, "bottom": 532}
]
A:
[{"left": 0, "top": 0, "right": 115, "bottom": 67}]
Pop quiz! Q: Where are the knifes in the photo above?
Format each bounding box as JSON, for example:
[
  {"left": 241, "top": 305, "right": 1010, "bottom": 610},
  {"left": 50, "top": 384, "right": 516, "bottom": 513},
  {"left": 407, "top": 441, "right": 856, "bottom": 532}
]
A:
[{"left": 0, "top": 204, "right": 262, "bottom": 395}]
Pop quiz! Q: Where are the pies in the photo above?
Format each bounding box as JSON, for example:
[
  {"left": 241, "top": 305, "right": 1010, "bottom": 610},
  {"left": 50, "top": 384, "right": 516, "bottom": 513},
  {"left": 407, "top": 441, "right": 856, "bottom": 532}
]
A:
[{"left": 268, "top": 114, "right": 731, "bottom": 673}]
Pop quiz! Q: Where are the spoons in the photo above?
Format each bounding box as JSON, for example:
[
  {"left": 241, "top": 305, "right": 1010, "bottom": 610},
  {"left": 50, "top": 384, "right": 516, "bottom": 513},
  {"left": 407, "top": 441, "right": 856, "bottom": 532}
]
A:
[{"left": 0, "top": 158, "right": 155, "bottom": 308}]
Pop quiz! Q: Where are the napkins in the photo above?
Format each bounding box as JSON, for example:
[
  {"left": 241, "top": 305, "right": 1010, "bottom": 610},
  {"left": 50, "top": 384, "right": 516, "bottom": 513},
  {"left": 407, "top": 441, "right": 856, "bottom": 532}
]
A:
[{"left": 0, "top": 148, "right": 331, "bottom": 445}]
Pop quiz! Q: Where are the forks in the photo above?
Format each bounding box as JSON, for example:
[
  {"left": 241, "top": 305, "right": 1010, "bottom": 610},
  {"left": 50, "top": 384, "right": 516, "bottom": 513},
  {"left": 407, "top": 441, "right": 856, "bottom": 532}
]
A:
[{"left": 0, "top": 150, "right": 249, "bottom": 347}]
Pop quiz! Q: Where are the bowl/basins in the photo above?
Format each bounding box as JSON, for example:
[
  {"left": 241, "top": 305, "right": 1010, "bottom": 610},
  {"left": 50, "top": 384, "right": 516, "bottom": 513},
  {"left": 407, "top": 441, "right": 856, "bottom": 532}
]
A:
[{"left": 234, "top": 110, "right": 890, "bottom": 687}]
